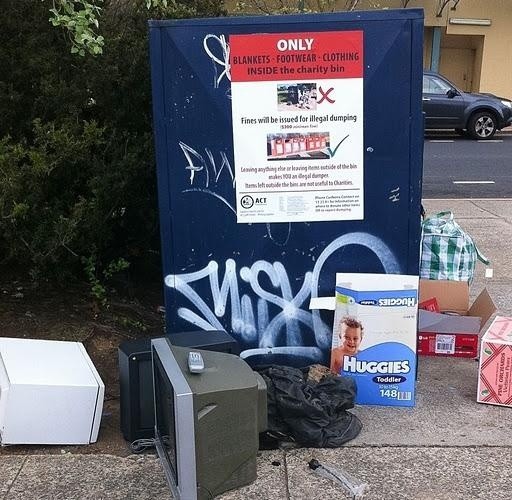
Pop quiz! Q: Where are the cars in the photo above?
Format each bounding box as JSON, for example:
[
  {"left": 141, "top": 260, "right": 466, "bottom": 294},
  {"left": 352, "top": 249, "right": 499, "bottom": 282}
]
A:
[{"left": 422, "top": 71, "right": 512, "bottom": 140}]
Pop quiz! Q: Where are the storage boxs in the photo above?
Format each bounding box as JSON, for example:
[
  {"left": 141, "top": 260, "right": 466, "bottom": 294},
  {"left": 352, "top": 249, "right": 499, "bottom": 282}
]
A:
[{"left": 419, "top": 279, "right": 512, "bottom": 407}]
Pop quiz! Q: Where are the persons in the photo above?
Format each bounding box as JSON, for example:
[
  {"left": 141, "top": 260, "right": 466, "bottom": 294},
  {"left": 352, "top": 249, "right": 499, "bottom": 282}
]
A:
[{"left": 330, "top": 319, "right": 364, "bottom": 374}]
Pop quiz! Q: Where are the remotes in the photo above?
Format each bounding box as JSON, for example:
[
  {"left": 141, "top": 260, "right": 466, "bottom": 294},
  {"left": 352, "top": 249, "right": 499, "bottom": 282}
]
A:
[{"left": 188, "top": 352, "right": 204, "bottom": 373}]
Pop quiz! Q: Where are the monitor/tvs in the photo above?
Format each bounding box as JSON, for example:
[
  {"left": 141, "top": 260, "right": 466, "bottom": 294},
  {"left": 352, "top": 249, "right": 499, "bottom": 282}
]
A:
[
  {"left": 150, "top": 338, "right": 268, "bottom": 500},
  {"left": 118, "top": 330, "right": 240, "bottom": 445}
]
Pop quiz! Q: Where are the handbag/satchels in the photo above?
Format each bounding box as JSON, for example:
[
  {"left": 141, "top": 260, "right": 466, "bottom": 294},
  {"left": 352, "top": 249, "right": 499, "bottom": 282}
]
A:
[{"left": 419, "top": 211, "right": 490, "bottom": 289}]
